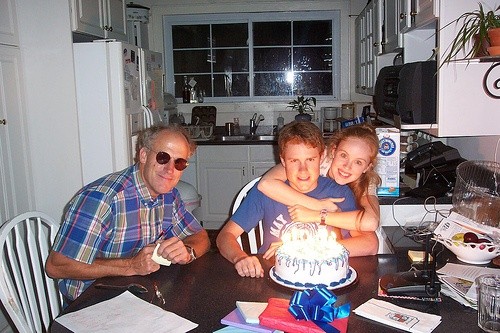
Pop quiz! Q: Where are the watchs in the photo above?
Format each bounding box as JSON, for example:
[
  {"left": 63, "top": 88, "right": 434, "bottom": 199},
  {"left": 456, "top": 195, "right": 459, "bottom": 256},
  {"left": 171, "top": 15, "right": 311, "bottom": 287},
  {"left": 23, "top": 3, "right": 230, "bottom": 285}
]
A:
[{"left": 185, "top": 245, "right": 197, "bottom": 264}]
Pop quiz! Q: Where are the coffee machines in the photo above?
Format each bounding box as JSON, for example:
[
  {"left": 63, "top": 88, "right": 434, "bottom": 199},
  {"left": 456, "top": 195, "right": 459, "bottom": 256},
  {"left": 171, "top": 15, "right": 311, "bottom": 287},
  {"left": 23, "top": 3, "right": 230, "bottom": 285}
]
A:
[{"left": 321, "top": 106, "right": 341, "bottom": 138}]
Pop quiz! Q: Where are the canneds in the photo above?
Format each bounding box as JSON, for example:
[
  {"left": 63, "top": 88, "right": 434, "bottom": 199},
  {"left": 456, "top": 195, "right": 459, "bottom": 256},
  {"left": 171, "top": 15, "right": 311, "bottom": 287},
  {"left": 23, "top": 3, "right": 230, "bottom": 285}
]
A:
[{"left": 341, "top": 103, "right": 354, "bottom": 120}]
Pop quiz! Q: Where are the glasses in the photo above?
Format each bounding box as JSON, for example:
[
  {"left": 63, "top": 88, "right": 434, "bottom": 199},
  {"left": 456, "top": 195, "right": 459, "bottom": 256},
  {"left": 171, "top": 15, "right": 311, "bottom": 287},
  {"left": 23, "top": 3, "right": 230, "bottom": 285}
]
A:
[{"left": 148, "top": 146, "right": 189, "bottom": 171}]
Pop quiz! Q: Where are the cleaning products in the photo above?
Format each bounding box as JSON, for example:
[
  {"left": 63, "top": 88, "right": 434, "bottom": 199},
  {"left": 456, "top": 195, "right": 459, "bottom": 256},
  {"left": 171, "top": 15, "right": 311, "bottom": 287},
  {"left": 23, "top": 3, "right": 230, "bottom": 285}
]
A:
[{"left": 277, "top": 113, "right": 285, "bottom": 133}]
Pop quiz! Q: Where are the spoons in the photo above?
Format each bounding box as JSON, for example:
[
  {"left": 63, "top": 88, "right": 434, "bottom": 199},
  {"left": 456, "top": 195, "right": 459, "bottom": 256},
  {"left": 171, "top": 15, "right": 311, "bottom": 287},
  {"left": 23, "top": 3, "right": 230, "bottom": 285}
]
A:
[{"left": 94, "top": 283, "right": 149, "bottom": 293}]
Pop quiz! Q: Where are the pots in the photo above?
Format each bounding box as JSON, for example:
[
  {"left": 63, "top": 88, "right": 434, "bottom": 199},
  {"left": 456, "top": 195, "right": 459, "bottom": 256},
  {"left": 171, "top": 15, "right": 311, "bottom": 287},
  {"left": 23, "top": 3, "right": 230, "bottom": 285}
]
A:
[{"left": 125, "top": 1, "right": 152, "bottom": 25}]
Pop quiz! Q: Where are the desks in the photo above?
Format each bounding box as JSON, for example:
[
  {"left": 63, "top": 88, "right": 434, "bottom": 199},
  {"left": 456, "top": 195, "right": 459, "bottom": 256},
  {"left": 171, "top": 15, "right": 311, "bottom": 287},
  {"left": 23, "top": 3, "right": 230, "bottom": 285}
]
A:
[{"left": 49, "top": 225, "right": 500, "bottom": 333}]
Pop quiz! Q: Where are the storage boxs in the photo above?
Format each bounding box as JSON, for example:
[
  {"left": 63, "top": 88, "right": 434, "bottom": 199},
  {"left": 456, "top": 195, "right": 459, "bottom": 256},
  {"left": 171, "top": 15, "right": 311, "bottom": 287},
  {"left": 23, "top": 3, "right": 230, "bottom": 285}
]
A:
[{"left": 375, "top": 127, "right": 400, "bottom": 197}]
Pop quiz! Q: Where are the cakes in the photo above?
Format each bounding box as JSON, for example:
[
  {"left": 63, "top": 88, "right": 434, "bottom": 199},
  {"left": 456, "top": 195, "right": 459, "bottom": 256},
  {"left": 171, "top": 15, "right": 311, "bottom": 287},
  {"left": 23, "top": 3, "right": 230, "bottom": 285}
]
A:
[{"left": 272, "top": 238, "right": 351, "bottom": 288}]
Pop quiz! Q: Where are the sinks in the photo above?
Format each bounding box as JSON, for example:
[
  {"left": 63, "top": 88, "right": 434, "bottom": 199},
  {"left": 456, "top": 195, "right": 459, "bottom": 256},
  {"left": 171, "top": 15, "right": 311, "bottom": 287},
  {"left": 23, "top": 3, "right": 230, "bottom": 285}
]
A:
[
  {"left": 218, "top": 135, "right": 252, "bottom": 141},
  {"left": 256, "top": 136, "right": 279, "bottom": 141}
]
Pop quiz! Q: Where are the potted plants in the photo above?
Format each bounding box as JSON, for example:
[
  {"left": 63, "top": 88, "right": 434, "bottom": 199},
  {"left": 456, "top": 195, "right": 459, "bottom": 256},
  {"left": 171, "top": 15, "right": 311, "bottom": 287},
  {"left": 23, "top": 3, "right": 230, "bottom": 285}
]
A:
[
  {"left": 286, "top": 94, "right": 318, "bottom": 121},
  {"left": 425, "top": 0, "right": 500, "bottom": 76}
]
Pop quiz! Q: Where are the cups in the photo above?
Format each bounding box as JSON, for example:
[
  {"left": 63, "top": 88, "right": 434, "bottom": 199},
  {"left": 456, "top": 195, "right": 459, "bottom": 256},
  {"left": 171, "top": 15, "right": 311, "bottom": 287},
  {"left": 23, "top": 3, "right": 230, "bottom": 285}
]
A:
[
  {"left": 225, "top": 123, "right": 233, "bottom": 132},
  {"left": 234, "top": 118, "right": 239, "bottom": 127},
  {"left": 475, "top": 274, "right": 500, "bottom": 333}
]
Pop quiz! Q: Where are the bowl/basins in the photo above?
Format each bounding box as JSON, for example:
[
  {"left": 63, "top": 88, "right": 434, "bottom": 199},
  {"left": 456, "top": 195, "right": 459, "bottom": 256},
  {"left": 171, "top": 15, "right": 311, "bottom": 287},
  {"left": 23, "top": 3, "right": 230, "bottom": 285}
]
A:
[{"left": 452, "top": 160, "right": 500, "bottom": 229}]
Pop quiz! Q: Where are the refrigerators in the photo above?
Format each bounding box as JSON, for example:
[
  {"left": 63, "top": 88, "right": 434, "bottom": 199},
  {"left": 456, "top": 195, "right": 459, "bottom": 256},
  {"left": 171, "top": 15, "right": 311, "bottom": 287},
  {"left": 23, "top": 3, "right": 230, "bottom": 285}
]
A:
[{"left": 72, "top": 38, "right": 165, "bottom": 187}]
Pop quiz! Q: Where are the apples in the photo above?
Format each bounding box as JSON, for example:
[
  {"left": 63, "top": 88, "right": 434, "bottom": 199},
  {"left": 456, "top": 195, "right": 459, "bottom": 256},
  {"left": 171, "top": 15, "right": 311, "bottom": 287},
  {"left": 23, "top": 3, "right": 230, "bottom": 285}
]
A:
[{"left": 463, "top": 232, "right": 493, "bottom": 252}]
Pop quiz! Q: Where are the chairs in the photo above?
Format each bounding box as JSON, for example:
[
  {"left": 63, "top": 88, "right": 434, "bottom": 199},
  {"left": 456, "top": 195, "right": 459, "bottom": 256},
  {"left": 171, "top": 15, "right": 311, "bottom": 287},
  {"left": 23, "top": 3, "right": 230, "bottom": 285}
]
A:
[
  {"left": 0, "top": 211, "right": 62, "bottom": 333},
  {"left": 232, "top": 175, "right": 264, "bottom": 254}
]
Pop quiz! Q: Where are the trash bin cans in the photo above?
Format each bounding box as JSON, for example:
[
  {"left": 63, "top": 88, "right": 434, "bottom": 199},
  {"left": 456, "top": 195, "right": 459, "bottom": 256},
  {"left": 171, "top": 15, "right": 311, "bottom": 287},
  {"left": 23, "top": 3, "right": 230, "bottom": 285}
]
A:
[{"left": 175, "top": 180, "right": 202, "bottom": 214}]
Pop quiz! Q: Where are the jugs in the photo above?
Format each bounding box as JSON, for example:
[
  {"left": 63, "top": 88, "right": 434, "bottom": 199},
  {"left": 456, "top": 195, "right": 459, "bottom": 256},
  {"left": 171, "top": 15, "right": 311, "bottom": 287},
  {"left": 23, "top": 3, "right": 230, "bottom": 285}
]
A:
[{"left": 324, "top": 119, "right": 338, "bottom": 132}]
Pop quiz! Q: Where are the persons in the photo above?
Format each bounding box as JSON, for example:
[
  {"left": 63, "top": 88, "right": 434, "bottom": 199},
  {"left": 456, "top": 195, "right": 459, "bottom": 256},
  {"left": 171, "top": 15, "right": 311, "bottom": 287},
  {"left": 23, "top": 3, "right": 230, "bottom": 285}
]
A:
[
  {"left": 257, "top": 121, "right": 382, "bottom": 232},
  {"left": 45, "top": 125, "right": 211, "bottom": 310},
  {"left": 215, "top": 121, "right": 380, "bottom": 278}
]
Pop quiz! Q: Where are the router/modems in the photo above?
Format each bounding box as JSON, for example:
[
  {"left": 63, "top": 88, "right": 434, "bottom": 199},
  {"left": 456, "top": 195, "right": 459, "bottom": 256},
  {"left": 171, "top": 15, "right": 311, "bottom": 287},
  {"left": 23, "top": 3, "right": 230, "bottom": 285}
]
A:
[{"left": 379, "top": 236, "right": 442, "bottom": 298}]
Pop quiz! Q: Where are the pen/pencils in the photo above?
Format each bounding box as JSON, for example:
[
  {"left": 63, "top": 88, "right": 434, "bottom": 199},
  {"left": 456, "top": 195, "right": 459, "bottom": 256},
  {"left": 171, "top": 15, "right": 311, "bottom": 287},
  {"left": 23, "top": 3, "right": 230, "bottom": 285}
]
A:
[
  {"left": 159, "top": 224, "right": 173, "bottom": 239},
  {"left": 158, "top": 228, "right": 166, "bottom": 239}
]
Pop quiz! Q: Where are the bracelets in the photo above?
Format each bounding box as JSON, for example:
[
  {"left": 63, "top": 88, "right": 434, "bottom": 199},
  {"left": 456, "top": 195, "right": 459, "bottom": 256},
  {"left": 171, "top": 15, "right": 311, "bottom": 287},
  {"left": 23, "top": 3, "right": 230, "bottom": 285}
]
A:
[{"left": 319, "top": 210, "right": 327, "bottom": 227}]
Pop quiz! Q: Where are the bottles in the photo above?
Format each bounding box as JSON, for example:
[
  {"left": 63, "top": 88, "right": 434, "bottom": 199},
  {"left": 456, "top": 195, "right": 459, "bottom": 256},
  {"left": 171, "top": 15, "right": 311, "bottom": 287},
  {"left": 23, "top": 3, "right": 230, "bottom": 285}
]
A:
[
  {"left": 341, "top": 104, "right": 354, "bottom": 120},
  {"left": 178, "top": 112, "right": 185, "bottom": 124},
  {"left": 277, "top": 113, "right": 284, "bottom": 133},
  {"left": 183, "top": 76, "right": 203, "bottom": 103}
]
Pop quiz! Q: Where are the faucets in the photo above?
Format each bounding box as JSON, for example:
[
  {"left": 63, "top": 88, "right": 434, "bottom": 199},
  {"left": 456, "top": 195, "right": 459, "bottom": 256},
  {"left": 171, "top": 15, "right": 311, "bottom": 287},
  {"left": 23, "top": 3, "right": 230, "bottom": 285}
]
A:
[{"left": 248, "top": 112, "right": 265, "bottom": 133}]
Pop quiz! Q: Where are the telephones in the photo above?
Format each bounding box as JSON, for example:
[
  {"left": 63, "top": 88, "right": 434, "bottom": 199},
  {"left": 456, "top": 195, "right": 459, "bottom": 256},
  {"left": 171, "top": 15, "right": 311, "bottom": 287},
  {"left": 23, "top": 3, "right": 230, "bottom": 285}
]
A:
[{"left": 406, "top": 141, "right": 463, "bottom": 173}]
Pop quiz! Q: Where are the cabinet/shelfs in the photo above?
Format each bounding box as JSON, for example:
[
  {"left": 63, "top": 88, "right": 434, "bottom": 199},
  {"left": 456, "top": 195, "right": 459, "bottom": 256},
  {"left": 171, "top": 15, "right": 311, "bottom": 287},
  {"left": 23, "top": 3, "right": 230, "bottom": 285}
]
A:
[
  {"left": 354, "top": 0, "right": 500, "bottom": 137},
  {"left": 195, "top": 145, "right": 281, "bottom": 228},
  {"left": 69, "top": 0, "right": 148, "bottom": 49}
]
